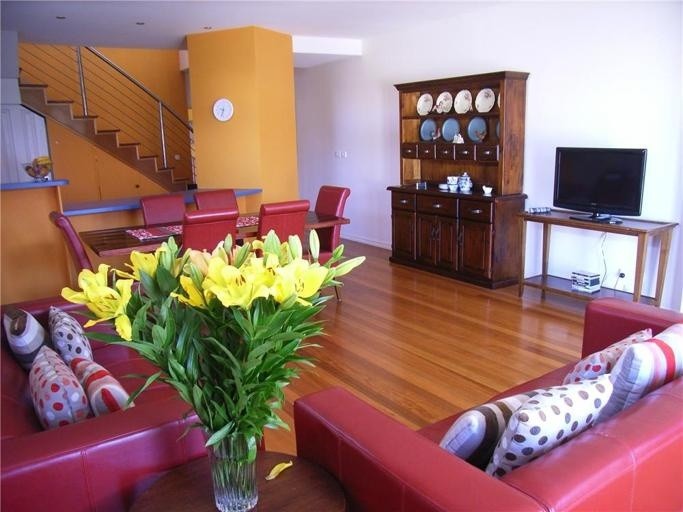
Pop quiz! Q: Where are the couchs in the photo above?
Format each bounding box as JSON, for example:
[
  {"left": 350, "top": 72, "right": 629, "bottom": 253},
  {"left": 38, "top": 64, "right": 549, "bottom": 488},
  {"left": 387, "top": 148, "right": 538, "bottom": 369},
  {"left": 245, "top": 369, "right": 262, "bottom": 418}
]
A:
[
  {"left": 294, "top": 297, "right": 683, "bottom": 512},
  {"left": 0, "top": 297, "right": 265, "bottom": 512}
]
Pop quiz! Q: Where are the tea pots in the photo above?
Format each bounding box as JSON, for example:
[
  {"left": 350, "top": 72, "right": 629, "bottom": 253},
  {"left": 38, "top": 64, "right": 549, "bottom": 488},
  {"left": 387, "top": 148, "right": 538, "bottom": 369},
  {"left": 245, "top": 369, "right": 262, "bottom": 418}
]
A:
[{"left": 457, "top": 171, "right": 473, "bottom": 193}]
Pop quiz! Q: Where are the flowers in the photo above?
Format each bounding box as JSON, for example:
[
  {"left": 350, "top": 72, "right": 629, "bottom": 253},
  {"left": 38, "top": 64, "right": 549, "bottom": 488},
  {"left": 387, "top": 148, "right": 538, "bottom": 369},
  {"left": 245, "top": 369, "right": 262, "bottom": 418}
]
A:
[{"left": 60, "top": 228, "right": 366, "bottom": 496}]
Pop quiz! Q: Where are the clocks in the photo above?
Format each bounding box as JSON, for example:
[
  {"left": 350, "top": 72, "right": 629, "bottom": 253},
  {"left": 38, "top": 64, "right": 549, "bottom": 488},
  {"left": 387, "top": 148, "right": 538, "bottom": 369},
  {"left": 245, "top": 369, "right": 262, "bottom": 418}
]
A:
[{"left": 212, "top": 98, "right": 233, "bottom": 122}]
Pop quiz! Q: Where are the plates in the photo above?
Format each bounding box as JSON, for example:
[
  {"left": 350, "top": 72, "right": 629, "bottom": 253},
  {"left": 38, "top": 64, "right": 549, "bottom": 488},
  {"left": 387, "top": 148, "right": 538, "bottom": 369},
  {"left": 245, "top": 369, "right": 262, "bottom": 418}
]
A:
[{"left": 416, "top": 86, "right": 501, "bottom": 144}]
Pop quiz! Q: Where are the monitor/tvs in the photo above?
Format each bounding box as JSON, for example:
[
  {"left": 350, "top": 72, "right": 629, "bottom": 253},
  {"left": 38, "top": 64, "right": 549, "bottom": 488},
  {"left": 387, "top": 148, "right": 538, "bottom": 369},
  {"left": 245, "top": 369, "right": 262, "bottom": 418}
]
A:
[{"left": 553, "top": 146, "right": 649, "bottom": 223}]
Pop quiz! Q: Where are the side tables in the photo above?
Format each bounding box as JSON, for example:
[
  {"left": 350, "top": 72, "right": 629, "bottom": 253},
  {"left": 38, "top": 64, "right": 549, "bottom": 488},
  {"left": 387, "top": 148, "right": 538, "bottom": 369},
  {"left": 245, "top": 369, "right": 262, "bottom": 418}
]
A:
[{"left": 131, "top": 452, "right": 348, "bottom": 512}]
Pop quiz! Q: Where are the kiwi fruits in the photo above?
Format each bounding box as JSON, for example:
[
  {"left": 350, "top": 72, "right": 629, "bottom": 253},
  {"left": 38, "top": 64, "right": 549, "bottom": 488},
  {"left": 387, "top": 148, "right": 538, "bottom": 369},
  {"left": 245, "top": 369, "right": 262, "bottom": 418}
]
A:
[
  {"left": 26, "top": 166, "right": 36, "bottom": 178},
  {"left": 39, "top": 166, "right": 49, "bottom": 177}
]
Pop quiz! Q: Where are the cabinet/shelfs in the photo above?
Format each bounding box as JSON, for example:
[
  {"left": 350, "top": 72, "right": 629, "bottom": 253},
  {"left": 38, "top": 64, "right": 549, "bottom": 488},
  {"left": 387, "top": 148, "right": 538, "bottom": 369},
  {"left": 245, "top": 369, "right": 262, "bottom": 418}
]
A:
[{"left": 387, "top": 70, "right": 530, "bottom": 289}]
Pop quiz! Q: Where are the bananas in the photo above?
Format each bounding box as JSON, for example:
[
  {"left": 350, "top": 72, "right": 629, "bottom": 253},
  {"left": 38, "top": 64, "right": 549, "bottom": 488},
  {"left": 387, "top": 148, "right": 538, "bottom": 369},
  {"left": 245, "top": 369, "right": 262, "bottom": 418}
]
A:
[{"left": 31, "top": 156, "right": 52, "bottom": 167}]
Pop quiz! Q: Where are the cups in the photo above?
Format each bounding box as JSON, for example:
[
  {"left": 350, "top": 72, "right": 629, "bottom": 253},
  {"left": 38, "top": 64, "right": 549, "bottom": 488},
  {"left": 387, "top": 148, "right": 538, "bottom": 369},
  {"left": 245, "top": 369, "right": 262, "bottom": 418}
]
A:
[
  {"left": 436, "top": 171, "right": 458, "bottom": 192},
  {"left": 482, "top": 186, "right": 492, "bottom": 196}
]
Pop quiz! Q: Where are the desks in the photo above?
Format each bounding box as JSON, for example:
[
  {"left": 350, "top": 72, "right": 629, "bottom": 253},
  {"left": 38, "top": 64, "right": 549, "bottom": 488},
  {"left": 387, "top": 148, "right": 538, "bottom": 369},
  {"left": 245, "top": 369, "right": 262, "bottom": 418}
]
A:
[{"left": 514, "top": 210, "right": 679, "bottom": 307}]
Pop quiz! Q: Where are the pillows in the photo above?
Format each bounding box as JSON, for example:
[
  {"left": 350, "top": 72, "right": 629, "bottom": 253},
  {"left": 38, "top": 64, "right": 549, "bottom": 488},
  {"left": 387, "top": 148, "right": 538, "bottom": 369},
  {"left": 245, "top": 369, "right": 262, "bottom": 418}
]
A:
[
  {"left": 3, "top": 305, "right": 134, "bottom": 430},
  {"left": 440, "top": 322, "right": 683, "bottom": 479}
]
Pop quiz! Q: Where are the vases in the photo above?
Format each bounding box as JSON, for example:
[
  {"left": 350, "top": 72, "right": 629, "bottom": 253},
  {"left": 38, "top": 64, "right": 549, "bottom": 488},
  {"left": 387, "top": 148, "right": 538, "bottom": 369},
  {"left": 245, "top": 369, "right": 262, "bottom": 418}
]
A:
[{"left": 204, "top": 425, "right": 258, "bottom": 512}]
